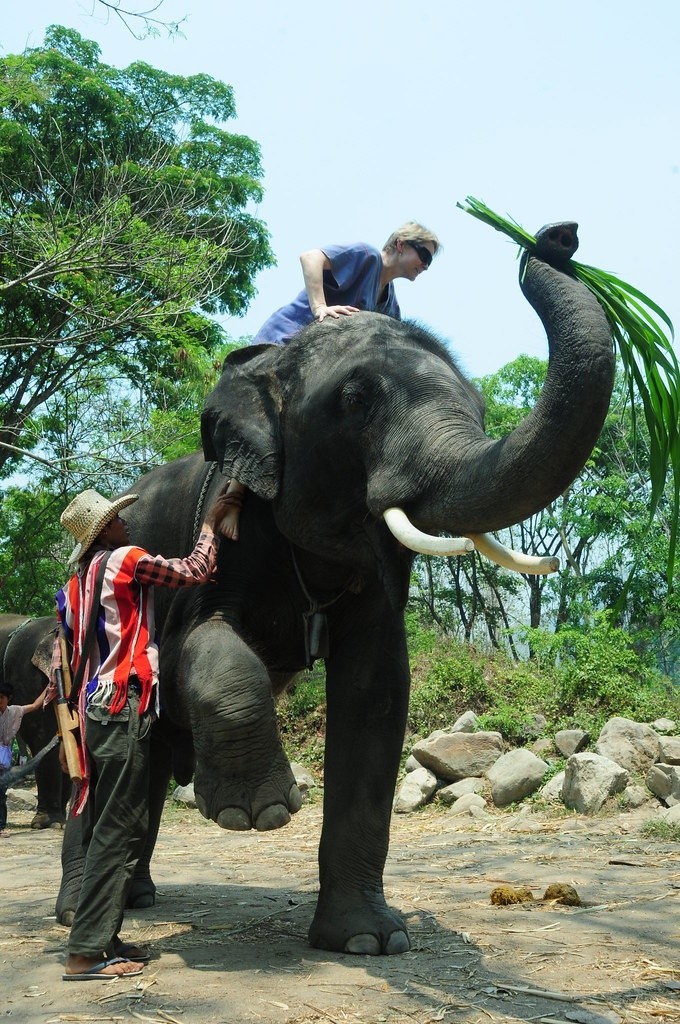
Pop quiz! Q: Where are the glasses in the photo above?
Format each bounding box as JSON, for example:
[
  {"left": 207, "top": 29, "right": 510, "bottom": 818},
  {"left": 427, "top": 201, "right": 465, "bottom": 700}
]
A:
[{"left": 406, "top": 240, "right": 432, "bottom": 267}]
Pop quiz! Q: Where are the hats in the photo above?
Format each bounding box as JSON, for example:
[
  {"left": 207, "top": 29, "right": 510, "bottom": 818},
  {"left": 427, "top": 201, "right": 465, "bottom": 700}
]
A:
[{"left": 60, "top": 489, "right": 139, "bottom": 565}]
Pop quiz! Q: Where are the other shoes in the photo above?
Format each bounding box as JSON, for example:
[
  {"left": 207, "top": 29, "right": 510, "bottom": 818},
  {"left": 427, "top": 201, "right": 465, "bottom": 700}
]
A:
[{"left": 0, "top": 827, "right": 10, "bottom": 837}]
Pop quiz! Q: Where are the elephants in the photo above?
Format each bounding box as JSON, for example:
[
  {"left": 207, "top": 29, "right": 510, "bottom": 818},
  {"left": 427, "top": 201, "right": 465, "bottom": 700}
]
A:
[{"left": 2, "top": 221, "right": 615, "bottom": 958}]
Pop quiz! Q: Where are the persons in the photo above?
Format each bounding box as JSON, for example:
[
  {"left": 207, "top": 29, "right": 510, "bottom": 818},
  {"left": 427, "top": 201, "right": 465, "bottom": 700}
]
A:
[
  {"left": 0, "top": 681, "right": 52, "bottom": 771},
  {"left": 53, "top": 479, "right": 242, "bottom": 981},
  {"left": 216, "top": 220, "right": 442, "bottom": 542}
]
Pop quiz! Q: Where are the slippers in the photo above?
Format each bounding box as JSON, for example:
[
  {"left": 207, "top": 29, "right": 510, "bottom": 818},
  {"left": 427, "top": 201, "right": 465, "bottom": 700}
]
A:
[
  {"left": 62, "top": 957, "right": 142, "bottom": 980},
  {"left": 112, "top": 944, "right": 151, "bottom": 961}
]
